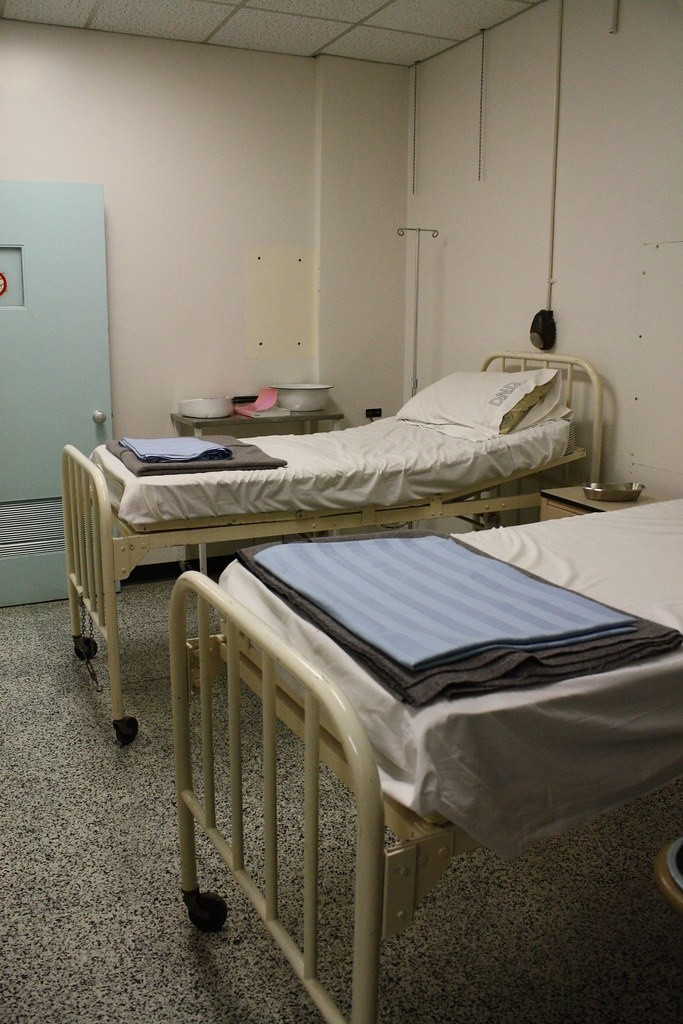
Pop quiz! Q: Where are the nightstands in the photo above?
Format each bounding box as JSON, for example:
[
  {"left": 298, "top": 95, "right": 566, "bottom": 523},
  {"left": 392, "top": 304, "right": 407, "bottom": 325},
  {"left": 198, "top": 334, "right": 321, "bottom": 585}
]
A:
[{"left": 541, "top": 485, "right": 677, "bottom": 521}]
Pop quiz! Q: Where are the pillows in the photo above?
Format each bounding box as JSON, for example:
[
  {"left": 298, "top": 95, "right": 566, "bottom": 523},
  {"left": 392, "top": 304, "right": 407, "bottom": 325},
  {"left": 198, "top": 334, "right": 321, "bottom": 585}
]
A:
[{"left": 395, "top": 369, "right": 562, "bottom": 439}]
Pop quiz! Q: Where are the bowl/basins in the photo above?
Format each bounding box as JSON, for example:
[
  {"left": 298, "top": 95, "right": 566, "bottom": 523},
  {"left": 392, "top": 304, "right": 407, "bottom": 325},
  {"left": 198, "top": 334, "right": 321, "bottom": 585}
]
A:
[
  {"left": 582, "top": 481, "right": 646, "bottom": 502},
  {"left": 269, "top": 383, "right": 336, "bottom": 412}
]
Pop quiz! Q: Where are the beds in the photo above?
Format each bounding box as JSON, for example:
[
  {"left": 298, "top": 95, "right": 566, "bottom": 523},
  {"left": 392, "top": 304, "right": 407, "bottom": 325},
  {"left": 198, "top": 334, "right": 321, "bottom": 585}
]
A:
[
  {"left": 169, "top": 497, "right": 683, "bottom": 1024},
  {"left": 64, "top": 351, "right": 604, "bottom": 742}
]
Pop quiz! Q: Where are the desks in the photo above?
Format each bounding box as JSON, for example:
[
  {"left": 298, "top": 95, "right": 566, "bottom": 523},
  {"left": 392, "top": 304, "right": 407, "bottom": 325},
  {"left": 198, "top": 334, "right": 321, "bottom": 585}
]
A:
[{"left": 172, "top": 409, "right": 345, "bottom": 437}]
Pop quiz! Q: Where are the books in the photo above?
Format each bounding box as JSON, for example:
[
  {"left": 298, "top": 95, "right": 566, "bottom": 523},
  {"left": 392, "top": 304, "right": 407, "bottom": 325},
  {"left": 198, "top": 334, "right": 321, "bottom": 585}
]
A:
[{"left": 234, "top": 387, "right": 291, "bottom": 419}]
